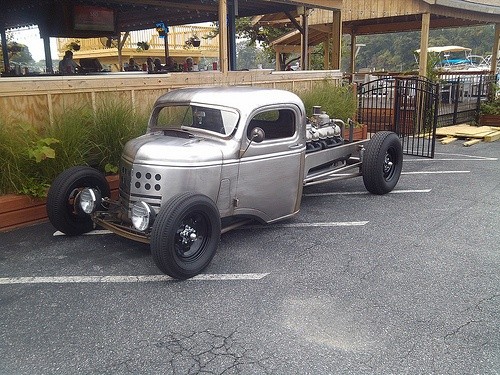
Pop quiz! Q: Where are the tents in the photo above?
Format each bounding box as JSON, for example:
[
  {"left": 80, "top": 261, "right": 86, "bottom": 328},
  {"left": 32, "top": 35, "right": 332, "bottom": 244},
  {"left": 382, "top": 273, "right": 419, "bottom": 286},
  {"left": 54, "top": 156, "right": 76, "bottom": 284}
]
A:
[{"left": 413, "top": 46, "right": 472, "bottom": 68}]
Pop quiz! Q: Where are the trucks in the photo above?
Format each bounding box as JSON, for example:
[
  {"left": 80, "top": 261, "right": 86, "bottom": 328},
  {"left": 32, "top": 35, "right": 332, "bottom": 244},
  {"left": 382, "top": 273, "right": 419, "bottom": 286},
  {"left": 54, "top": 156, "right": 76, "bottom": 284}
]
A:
[{"left": 46, "top": 87, "right": 403, "bottom": 279}]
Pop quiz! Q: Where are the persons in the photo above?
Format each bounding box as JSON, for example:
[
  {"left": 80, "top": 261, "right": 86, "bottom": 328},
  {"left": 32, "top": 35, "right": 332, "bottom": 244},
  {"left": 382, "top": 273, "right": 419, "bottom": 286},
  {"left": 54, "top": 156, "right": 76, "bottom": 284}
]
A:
[
  {"left": 123, "top": 57, "right": 195, "bottom": 73},
  {"left": 285, "top": 65, "right": 294, "bottom": 71},
  {"left": 59, "top": 51, "right": 82, "bottom": 74}
]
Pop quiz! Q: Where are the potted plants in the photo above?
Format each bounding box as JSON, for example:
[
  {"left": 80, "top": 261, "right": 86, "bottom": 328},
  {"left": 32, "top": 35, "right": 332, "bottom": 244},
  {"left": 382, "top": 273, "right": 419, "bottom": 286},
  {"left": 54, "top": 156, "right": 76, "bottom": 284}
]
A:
[
  {"left": 65, "top": 40, "right": 81, "bottom": 51},
  {"left": 249, "top": 29, "right": 270, "bottom": 46},
  {"left": 10, "top": 41, "right": 25, "bottom": 52},
  {"left": 136, "top": 41, "right": 150, "bottom": 53},
  {"left": 182, "top": 35, "right": 201, "bottom": 51}
]
